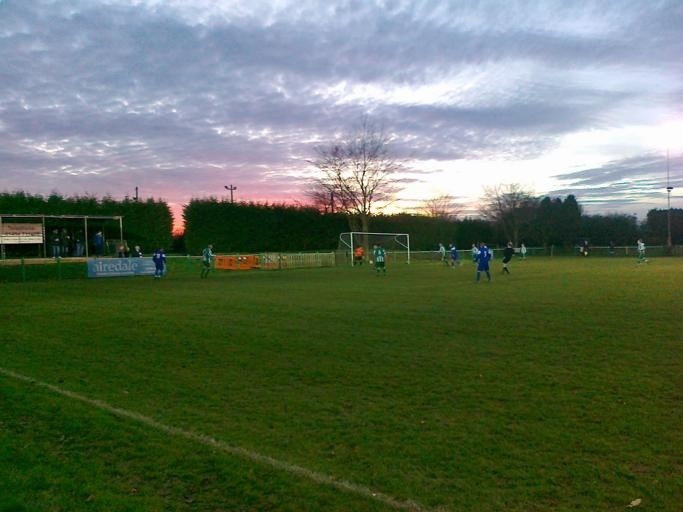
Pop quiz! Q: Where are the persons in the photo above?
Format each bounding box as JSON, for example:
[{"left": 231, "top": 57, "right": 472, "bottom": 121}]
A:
[
  {"left": 371, "top": 245, "right": 377, "bottom": 270},
  {"left": 373, "top": 244, "right": 388, "bottom": 274},
  {"left": 354, "top": 245, "right": 364, "bottom": 265},
  {"left": 94, "top": 231, "right": 103, "bottom": 258},
  {"left": 502, "top": 244, "right": 514, "bottom": 275},
  {"left": 508, "top": 240, "right": 514, "bottom": 256},
  {"left": 475, "top": 239, "right": 492, "bottom": 284},
  {"left": 448, "top": 241, "right": 462, "bottom": 267},
  {"left": 635, "top": 238, "right": 648, "bottom": 264},
  {"left": 152, "top": 247, "right": 167, "bottom": 280},
  {"left": 200, "top": 243, "right": 216, "bottom": 279},
  {"left": 132, "top": 245, "right": 144, "bottom": 258},
  {"left": 608, "top": 240, "right": 619, "bottom": 256},
  {"left": 58, "top": 229, "right": 70, "bottom": 255},
  {"left": 75, "top": 228, "right": 84, "bottom": 256},
  {"left": 437, "top": 242, "right": 448, "bottom": 266},
  {"left": 519, "top": 244, "right": 526, "bottom": 260},
  {"left": 119, "top": 246, "right": 128, "bottom": 258},
  {"left": 50, "top": 228, "right": 62, "bottom": 259},
  {"left": 471, "top": 244, "right": 479, "bottom": 272}
]
